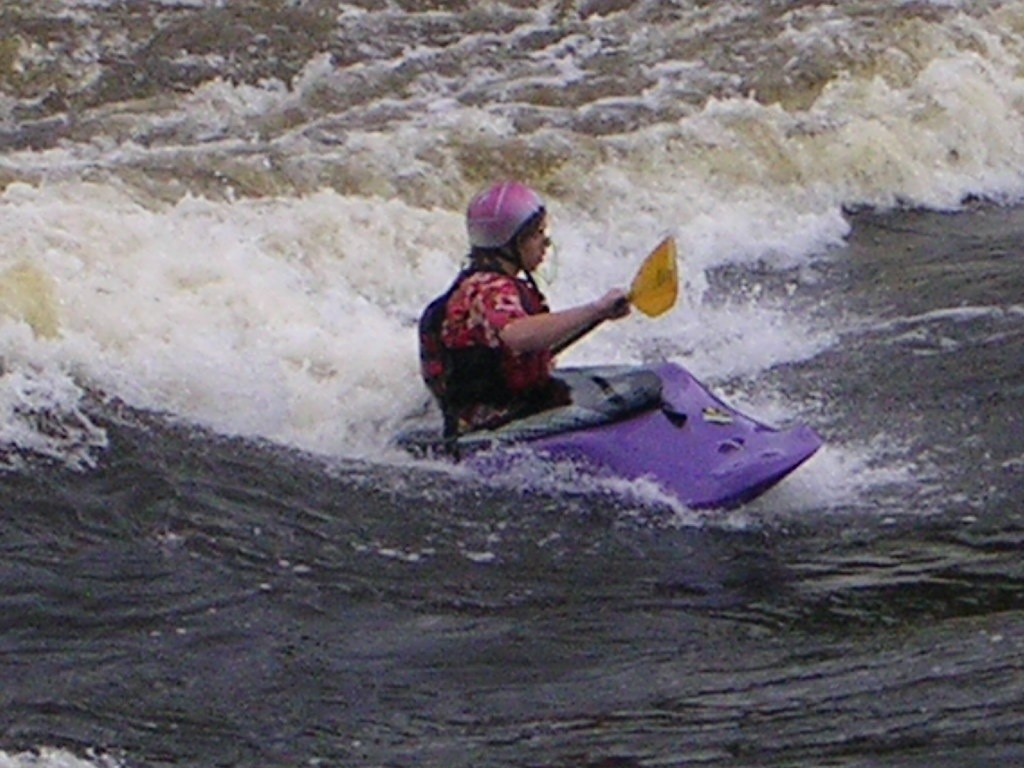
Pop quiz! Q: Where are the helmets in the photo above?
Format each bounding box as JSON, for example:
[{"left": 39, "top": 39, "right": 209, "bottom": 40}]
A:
[{"left": 464, "top": 177, "right": 543, "bottom": 250}]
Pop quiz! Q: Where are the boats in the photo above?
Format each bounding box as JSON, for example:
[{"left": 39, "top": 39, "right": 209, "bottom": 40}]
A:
[{"left": 386, "top": 364, "right": 822, "bottom": 512}]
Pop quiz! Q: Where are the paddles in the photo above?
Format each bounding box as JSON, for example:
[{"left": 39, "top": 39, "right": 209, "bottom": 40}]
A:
[{"left": 545, "top": 237, "right": 679, "bottom": 366}]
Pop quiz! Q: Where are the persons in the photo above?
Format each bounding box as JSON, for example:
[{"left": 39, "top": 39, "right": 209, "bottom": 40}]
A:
[{"left": 418, "top": 183, "right": 627, "bottom": 430}]
